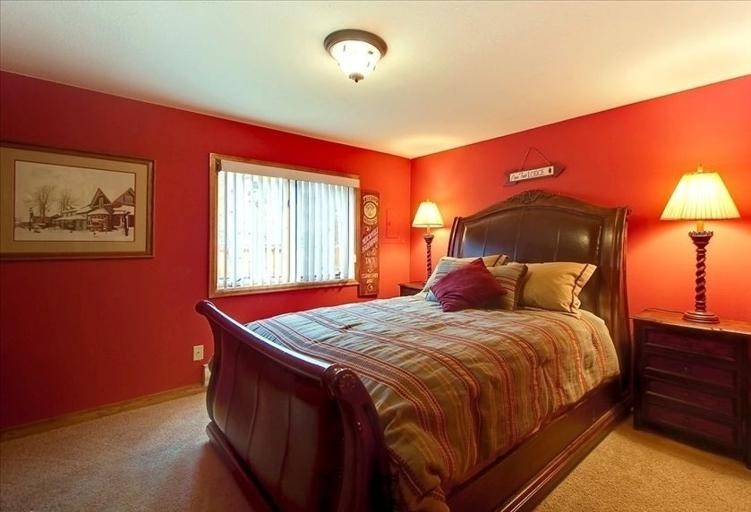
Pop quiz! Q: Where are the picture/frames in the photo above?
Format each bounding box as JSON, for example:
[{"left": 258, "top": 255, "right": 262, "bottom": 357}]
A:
[{"left": 0, "top": 140, "right": 155, "bottom": 263}]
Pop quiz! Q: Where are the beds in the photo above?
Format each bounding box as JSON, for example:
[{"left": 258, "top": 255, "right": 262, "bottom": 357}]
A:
[{"left": 195, "top": 187, "right": 633, "bottom": 512}]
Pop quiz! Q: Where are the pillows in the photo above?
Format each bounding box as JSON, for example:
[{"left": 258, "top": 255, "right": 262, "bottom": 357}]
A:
[{"left": 412, "top": 253, "right": 598, "bottom": 321}]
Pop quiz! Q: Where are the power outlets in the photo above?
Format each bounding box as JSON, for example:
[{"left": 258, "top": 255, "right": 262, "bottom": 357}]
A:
[{"left": 193, "top": 344, "right": 204, "bottom": 361}]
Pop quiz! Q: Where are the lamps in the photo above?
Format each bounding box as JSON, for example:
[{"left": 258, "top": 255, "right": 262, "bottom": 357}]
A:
[
  {"left": 411, "top": 199, "right": 444, "bottom": 286},
  {"left": 322, "top": 29, "right": 388, "bottom": 82},
  {"left": 657, "top": 166, "right": 741, "bottom": 324}
]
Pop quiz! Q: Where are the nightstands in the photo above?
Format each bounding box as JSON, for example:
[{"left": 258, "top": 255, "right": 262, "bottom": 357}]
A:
[
  {"left": 628, "top": 307, "right": 751, "bottom": 470},
  {"left": 397, "top": 279, "right": 425, "bottom": 297}
]
